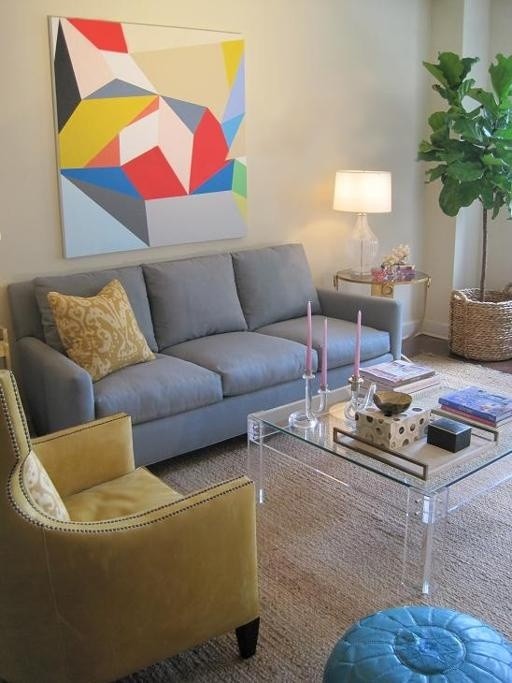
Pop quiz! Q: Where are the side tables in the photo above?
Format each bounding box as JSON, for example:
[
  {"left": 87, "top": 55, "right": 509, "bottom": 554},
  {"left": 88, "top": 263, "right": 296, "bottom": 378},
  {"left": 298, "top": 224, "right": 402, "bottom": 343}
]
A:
[{"left": 334, "top": 270, "right": 432, "bottom": 364}]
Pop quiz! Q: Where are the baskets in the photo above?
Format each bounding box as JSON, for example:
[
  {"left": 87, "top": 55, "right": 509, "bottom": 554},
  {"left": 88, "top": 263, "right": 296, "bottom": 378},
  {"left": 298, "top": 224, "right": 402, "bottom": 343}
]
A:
[{"left": 448, "top": 281, "right": 512, "bottom": 361}]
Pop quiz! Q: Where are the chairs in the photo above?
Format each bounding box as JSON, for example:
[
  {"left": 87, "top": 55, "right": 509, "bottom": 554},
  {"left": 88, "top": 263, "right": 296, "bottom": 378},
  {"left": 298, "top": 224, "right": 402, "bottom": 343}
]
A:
[{"left": 0, "top": 370, "right": 260, "bottom": 683}]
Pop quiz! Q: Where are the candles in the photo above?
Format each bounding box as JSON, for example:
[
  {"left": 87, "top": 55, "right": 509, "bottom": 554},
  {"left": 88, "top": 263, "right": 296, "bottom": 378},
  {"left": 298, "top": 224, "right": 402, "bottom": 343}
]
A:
[
  {"left": 320, "top": 317, "right": 328, "bottom": 391},
  {"left": 354, "top": 310, "right": 362, "bottom": 377}
]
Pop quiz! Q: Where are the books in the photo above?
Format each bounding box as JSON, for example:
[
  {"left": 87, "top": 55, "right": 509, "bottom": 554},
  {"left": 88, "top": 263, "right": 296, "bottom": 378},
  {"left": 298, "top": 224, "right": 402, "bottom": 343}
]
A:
[
  {"left": 429, "top": 384, "right": 511, "bottom": 441},
  {"left": 348, "top": 359, "right": 441, "bottom": 395}
]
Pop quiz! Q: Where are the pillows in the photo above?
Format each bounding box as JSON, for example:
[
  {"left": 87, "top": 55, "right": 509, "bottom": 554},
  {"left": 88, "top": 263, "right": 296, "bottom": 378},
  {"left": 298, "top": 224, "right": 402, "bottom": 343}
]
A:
[{"left": 46, "top": 280, "right": 155, "bottom": 382}]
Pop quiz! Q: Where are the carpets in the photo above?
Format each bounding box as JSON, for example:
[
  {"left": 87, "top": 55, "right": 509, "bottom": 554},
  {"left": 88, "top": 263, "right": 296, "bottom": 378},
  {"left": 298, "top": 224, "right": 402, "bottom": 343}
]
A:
[{"left": 118, "top": 353, "right": 512, "bottom": 683}]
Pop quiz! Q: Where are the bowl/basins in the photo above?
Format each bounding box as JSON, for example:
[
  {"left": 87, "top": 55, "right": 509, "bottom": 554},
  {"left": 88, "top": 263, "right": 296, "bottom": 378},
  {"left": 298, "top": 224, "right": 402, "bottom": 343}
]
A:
[{"left": 373, "top": 390, "right": 411, "bottom": 414}]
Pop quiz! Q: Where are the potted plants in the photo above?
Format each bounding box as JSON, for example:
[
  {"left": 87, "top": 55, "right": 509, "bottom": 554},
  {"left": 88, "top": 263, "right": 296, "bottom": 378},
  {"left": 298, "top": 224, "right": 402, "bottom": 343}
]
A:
[
  {"left": 415, "top": 50, "right": 512, "bottom": 362},
  {"left": 306, "top": 301, "right": 312, "bottom": 374}
]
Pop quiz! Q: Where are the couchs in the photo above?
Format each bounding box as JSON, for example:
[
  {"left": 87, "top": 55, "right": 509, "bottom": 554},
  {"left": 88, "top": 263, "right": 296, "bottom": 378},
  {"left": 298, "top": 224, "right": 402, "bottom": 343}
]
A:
[{"left": 7, "top": 280, "right": 403, "bottom": 467}]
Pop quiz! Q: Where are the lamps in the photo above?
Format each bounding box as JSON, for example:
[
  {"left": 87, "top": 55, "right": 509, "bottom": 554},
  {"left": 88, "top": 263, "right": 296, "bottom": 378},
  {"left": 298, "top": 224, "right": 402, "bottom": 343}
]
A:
[{"left": 333, "top": 170, "right": 392, "bottom": 278}]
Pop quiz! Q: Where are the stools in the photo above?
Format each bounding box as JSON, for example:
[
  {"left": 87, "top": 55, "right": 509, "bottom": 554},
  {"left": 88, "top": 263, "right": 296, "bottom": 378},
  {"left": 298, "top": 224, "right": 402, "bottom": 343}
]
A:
[{"left": 322, "top": 605, "right": 512, "bottom": 683}]
